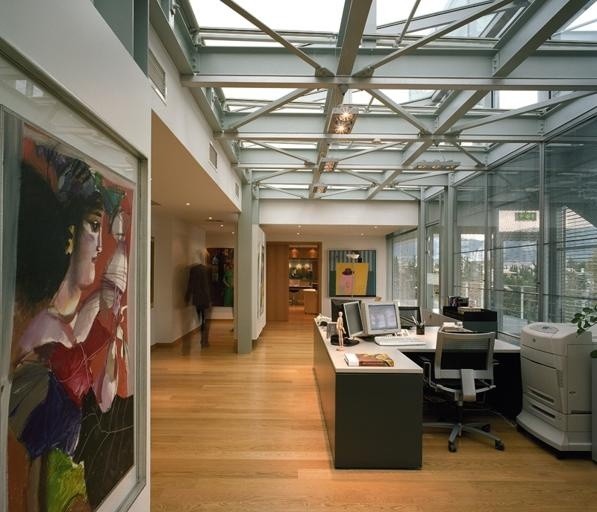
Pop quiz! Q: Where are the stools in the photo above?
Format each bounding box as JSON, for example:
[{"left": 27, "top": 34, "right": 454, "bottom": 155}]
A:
[{"left": 289, "top": 288, "right": 299, "bottom": 305}]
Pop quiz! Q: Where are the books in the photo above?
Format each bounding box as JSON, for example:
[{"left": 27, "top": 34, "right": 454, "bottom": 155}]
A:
[{"left": 343, "top": 353, "right": 394, "bottom": 367}]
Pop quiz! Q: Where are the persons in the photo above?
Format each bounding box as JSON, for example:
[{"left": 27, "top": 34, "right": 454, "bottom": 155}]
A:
[
  {"left": 223, "top": 254, "right": 235, "bottom": 331},
  {"left": 185, "top": 248, "right": 213, "bottom": 349},
  {"left": 15, "top": 151, "right": 134, "bottom": 505}
]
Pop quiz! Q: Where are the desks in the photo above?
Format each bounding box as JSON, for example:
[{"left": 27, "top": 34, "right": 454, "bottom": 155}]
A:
[{"left": 312, "top": 316, "right": 524, "bottom": 470}]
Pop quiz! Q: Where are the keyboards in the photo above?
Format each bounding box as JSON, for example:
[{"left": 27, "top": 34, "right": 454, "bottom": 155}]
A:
[{"left": 374, "top": 335, "right": 426, "bottom": 346}]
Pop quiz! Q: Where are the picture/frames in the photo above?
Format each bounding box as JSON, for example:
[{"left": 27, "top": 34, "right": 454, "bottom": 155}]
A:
[{"left": 0, "top": 36, "right": 149, "bottom": 512}]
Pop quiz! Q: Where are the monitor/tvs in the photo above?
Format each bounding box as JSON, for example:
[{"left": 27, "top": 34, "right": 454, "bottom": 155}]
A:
[
  {"left": 343, "top": 301, "right": 364, "bottom": 346},
  {"left": 364, "top": 301, "right": 401, "bottom": 335},
  {"left": 331, "top": 299, "right": 361, "bottom": 347}
]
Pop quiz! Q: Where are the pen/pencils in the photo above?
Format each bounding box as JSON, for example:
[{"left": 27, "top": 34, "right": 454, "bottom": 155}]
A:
[{"left": 411, "top": 315, "right": 425, "bottom": 334}]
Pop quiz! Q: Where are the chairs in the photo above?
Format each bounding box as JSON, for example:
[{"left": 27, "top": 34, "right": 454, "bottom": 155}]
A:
[{"left": 413, "top": 329, "right": 506, "bottom": 453}]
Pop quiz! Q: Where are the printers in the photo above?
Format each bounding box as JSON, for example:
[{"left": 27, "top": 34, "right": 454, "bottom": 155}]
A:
[{"left": 515, "top": 320, "right": 597, "bottom": 455}]
